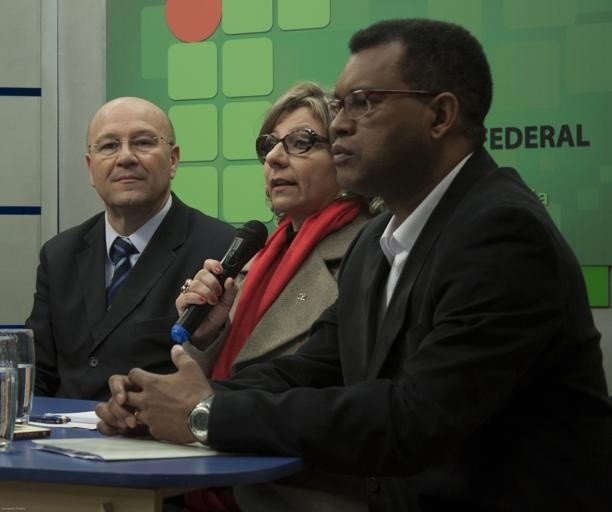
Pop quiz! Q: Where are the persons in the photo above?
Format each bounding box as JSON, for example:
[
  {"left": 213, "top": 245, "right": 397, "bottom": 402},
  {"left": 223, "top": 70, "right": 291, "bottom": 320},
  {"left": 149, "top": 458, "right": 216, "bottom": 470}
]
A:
[
  {"left": 97, "top": 17, "right": 612, "bottom": 512},
  {"left": 175, "top": 81, "right": 385, "bottom": 381},
  {"left": 17, "top": 97, "right": 237, "bottom": 400}
]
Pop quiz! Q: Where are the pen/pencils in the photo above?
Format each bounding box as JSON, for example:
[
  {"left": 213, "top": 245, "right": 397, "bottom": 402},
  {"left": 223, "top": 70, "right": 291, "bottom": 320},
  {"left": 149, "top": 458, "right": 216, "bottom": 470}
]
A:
[{"left": 29, "top": 415, "right": 71, "bottom": 424}]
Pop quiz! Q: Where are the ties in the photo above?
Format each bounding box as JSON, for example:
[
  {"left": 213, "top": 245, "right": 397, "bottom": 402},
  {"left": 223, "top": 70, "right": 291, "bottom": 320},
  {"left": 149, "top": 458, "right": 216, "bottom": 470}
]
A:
[{"left": 105, "top": 236, "right": 140, "bottom": 313}]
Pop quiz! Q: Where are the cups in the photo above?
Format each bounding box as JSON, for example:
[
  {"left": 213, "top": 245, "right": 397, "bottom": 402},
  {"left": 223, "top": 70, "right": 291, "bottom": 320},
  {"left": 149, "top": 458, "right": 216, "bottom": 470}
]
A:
[{"left": 0, "top": 328, "right": 36, "bottom": 452}]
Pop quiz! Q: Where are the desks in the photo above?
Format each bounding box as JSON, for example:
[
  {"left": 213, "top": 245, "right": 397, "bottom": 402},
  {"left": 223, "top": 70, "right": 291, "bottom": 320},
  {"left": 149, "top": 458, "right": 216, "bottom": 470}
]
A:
[{"left": 0, "top": 395, "right": 307, "bottom": 512}]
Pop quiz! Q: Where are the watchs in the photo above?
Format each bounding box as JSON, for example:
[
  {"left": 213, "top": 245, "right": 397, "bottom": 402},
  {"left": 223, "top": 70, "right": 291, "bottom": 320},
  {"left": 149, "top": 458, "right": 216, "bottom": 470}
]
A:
[{"left": 187, "top": 393, "right": 216, "bottom": 445}]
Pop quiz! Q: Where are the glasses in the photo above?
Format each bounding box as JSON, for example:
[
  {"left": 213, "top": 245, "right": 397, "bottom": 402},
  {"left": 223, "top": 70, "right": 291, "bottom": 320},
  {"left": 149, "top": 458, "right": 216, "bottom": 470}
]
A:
[
  {"left": 255, "top": 128, "right": 329, "bottom": 165},
  {"left": 329, "top": 89, "right": 434, "bottom": 120},
  {"left": 89, "top": 133, "right": 174, "bottom": 157}
]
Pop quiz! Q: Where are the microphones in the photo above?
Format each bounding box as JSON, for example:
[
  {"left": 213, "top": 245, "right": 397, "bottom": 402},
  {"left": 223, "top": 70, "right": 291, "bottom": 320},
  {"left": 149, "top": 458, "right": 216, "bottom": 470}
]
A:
[{"left": 170, "top": 220, "right": 268, "bottom": 345}]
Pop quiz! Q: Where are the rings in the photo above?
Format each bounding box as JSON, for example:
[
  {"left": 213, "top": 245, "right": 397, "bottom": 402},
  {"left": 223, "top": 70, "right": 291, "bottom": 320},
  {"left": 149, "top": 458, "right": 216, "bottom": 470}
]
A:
[
  {"left": 180, "top": 279, "right": 192, "bottom": 293},
  {"left": 134, "top": 411, "right": 141, "bottom": 424}
]
